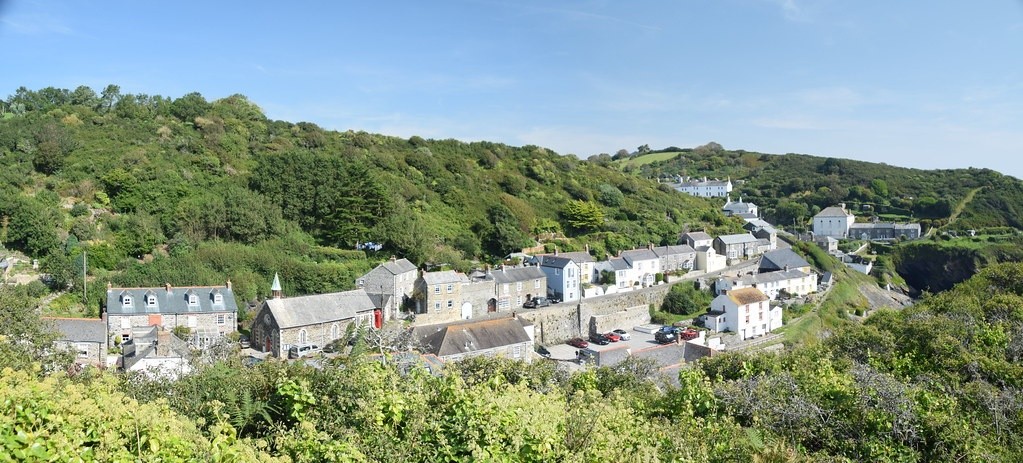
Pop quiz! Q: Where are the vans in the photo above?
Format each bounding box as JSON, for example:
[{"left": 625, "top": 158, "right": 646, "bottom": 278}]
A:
[{"left": 291, "top": 344, "right": 324, "bottom": 360}]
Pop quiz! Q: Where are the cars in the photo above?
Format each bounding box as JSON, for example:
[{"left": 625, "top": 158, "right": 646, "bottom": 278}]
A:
[
  {"left": 238, "top": 338, "right": 250, "bottom": 349},
  {"left": 570, "top": 338, "right": 588, "bottom": 348},
  {"left": 551, "top": 295, "right": 560, "bottom": 303},
  {"left": 613, "top": 329, "right": 630, "bottom": 340},
  {"left": 605, "top": 332, "right": 620, "bottom": 342},
  {"left": 326, "top": 339, "right": 346, "bottom": 353},
  {"left": 591, "top": 333, "right": 609, "bottom": 345},
  {"left": 526, "top": 296, "right": 551, "bottom": 309}
]
